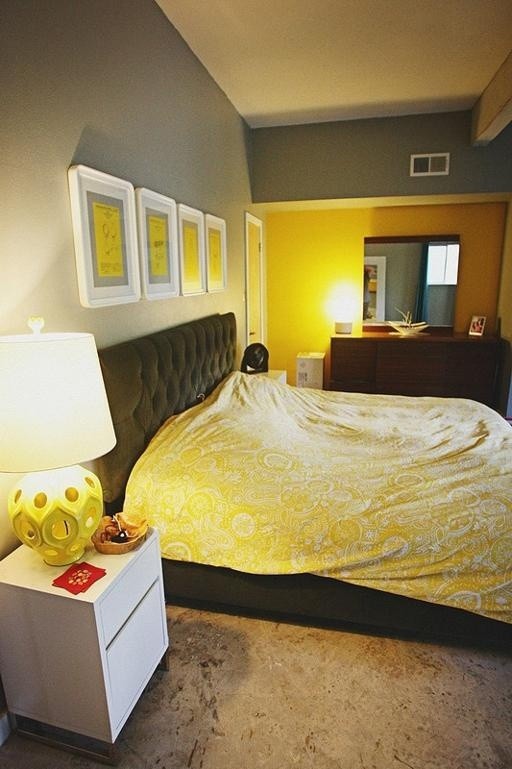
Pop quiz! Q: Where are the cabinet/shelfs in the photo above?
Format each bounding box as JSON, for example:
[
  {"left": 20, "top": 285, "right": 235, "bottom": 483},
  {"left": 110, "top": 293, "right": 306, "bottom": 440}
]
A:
[
  {"left": 325, "top": 332, "right": 499, "bottom": 411},
  {"left": 0, "top": 526, "right": 171, "bottom": 767}
]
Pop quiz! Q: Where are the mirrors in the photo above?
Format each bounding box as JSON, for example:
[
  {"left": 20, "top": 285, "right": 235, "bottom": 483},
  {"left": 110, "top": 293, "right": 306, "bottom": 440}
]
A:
[{"left": 363, "top": 235, "right": 460, "bottom": 336}]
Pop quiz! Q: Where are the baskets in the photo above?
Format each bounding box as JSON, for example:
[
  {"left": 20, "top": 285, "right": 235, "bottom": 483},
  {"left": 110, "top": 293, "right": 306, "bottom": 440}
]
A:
[{"left": 91, "top": 517, "right": 150, "bottom": 553}]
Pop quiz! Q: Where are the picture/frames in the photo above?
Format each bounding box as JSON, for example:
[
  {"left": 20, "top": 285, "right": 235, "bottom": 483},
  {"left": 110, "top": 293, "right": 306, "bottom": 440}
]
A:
[
  {"left": 68, "top": 164, "right": 228, "bottom": 308},
  {"left": 469, "top": 315, "right": 487, "bottom": 336}
]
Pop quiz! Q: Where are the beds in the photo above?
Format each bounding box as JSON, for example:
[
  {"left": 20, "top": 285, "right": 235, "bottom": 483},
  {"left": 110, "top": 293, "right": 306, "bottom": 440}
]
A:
[{"left": 85, "top": 312, "right": 511, "bottom": 644}]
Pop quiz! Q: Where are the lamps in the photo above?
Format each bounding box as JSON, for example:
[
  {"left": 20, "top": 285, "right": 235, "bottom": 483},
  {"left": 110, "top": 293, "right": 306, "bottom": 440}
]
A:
[{"left": 0, "top": 332, "right": 118, "bottom": 567}]
